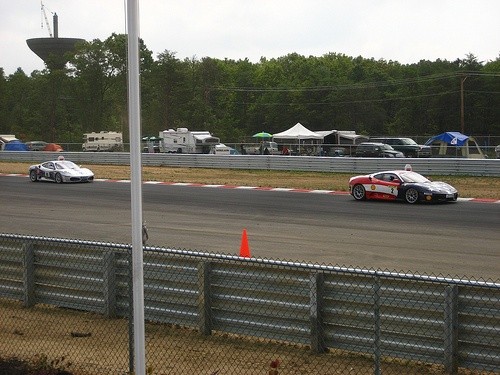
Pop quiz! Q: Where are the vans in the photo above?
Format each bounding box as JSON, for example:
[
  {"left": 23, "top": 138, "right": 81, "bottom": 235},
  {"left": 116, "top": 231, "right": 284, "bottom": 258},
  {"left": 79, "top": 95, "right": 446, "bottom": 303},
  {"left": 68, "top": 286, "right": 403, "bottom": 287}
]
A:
[
  {"left": 82, "top": 131, "right": 124, "bottom": 151},
  {"left": 26, "top": 141, "right": 48, "bottom": 151},
  {"left": 158, "top": 127, "right": 220, "bottom": 154},
  {"left": 245, "top": 141, "right": 278, "bottom": 157}
]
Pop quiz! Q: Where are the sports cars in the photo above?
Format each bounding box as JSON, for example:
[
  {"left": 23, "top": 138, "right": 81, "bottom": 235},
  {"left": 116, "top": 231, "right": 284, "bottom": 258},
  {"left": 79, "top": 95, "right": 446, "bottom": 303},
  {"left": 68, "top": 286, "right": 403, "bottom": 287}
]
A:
[
  {"left": 27, "top": 160, "right": 94, "bottom": 184},
  {"left": 347, "top": 170, "right": 460, "bottom": 204}
]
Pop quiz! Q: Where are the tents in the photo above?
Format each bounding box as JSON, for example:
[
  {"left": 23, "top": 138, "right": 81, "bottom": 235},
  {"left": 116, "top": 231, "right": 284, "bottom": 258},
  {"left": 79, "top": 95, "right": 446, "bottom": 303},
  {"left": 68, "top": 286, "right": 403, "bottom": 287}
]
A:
[{"left": 424, "top": 132, "right": 469, "bottom": 156}]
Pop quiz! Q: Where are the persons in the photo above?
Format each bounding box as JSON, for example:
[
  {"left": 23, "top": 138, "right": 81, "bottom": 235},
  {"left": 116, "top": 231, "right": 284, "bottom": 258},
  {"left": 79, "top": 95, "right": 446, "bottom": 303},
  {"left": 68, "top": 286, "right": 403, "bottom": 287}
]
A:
[
  {"left": 265, "top": 147, "right": 269, "bottom": 154},
  {"left": 281, "top": 145, "right": 288, "bottom": 155}
]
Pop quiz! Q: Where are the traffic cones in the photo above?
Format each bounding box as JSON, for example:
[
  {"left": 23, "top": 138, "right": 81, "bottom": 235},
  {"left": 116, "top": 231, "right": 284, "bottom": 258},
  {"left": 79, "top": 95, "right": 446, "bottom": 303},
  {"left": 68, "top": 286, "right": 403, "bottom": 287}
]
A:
[{"left": 240, "top": 227, "right": 251, "bottom": 257}]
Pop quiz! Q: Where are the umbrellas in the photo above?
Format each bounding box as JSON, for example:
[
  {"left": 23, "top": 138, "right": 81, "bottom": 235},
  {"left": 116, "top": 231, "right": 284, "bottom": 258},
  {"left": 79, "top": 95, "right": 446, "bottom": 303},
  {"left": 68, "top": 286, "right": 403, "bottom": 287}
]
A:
[
  {"left": 273, "top": 122, "right": 323, "bottom": 152},
  {"left": 252, "top": 131, "right": 273, "bottom": 137}
]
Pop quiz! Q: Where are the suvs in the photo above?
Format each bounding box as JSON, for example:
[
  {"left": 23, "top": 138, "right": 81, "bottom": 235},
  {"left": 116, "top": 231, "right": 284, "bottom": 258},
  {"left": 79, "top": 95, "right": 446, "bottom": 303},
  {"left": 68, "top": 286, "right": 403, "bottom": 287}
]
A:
[
  {"left": 371, "top": 138, "right": 432, "bottom": 158},
  {"left": 356, "top": 142, "right": 405, "bottom": 158}
]
